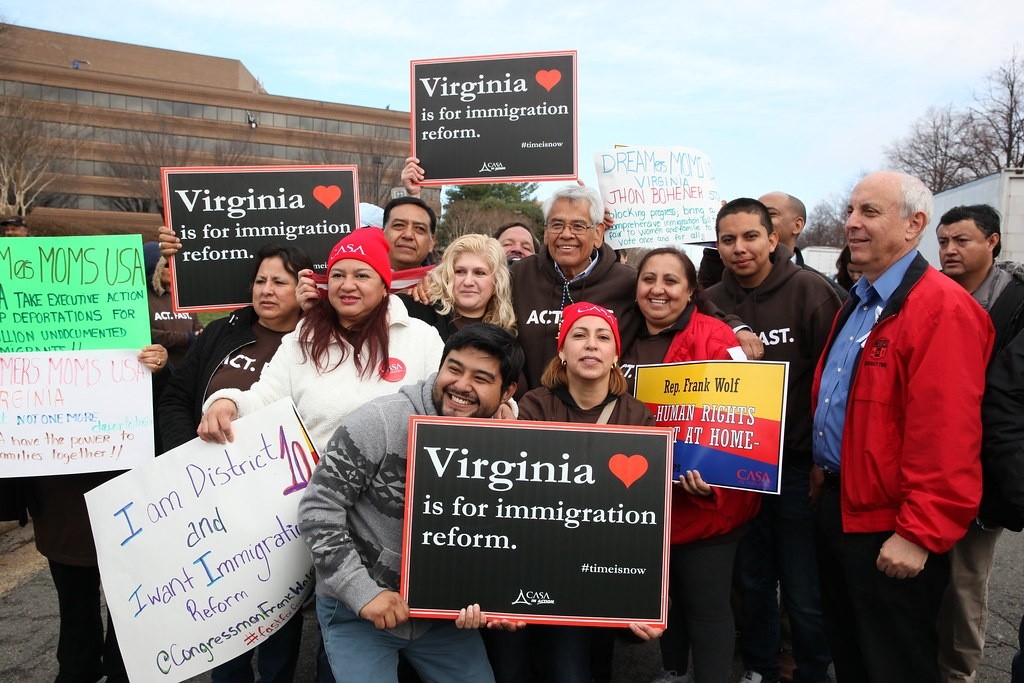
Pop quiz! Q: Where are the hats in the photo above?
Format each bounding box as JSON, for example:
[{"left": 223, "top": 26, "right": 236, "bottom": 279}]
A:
[
  {"left": 327, "top": 227, "right": 392, "bottom": 292},
  {"left": 558, "top": 300, "right": 620, "bottom": 360}
]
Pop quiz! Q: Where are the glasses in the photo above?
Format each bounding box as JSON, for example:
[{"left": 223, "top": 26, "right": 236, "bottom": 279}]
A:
[{"left": 545, "top": 217, "right": 597, "bottom": 235}]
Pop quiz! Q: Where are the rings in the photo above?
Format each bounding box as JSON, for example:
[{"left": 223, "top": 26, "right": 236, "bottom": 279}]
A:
[{"left": 759, "top": 352, "right": 763, "bottom": 356}]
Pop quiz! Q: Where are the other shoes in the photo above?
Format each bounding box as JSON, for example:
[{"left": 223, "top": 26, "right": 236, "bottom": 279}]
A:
[
  {"left": 650, "top": 666, "right": 692, "bottom": 683},
  {"left": 738, "top": 670, "right": 763, "bottom": 683}
]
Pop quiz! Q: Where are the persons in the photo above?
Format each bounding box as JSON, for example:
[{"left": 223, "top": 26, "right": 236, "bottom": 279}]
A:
[
  {"left": 156, "top": 245, "right": 313, "bottom": 682},
  {"left": 400, "top": 155, "right": 541, "bottom": 267},
  {"left": 383, "top": 197, "right": 436, "bottom": 272},
  {"left": 936, "top": 205, "right": 1024, "bottom": 682},
  {"left": 620, "top": 247, "right": 762, "bottom": 683},
  {"left": 487, "top": 302, "right": 656, "bottom": 682},
  {"left": 826, "top": 245, "right": 864, "bottom": 291},
  {"left": 813, "top": 172, "right": 995, "bottom": 682},
  {"left": 0, "top": 216, "right": 34, "bottom": 237},
  {"left": 393, "top": 234, "right": 519, "bottom": 343},
  {"left": 298, "top": 323, "right": 526, "bottom": 683},
  {"left": 158, "top": 201, "right": 384, "bottom": 255},
  {"left": 705, "top": 198, "right": 842, "bottom": 682},
  {"left": 698, "top": 192, "right": 850, "bottom": 304},
  {"left": 25, "top": 344, "right": 174, "bottom": 683},
  {"left": 405, "top": 185, "right": 764, "bottom": 403},
  {"left": 197, "top": 225, "right": 519, "bottom": 683},
  {"left": 142, "top": 241, "right": 204, "bottom": 347}
]
